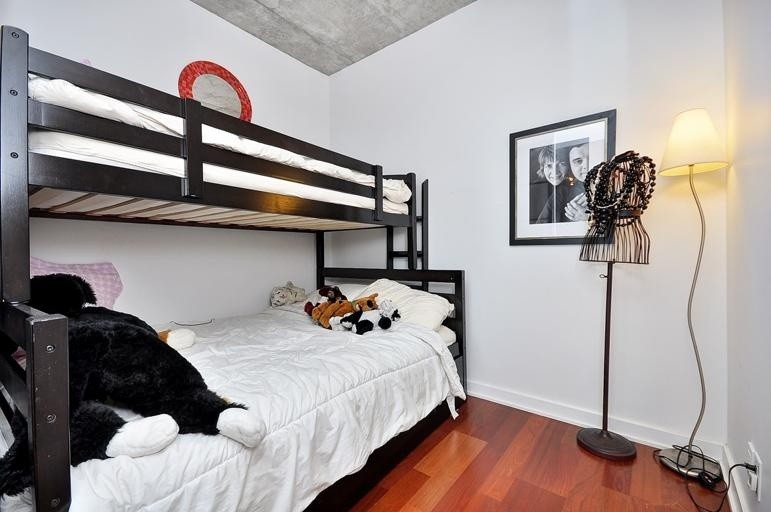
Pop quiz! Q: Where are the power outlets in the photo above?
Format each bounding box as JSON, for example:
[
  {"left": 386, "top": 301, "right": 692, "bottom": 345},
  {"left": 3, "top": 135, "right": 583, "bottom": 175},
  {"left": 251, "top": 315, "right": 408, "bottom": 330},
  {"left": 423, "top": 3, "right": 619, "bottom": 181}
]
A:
[{"left": 745, "top": 441, "right": 762, "bottom": 501}]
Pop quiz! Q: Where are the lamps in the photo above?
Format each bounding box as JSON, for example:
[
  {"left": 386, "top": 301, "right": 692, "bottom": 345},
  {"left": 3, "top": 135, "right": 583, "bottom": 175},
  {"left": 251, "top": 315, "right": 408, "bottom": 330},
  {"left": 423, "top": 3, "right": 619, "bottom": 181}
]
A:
[{"left": 658, "top": 108, "right": 730, "bottom": 483}]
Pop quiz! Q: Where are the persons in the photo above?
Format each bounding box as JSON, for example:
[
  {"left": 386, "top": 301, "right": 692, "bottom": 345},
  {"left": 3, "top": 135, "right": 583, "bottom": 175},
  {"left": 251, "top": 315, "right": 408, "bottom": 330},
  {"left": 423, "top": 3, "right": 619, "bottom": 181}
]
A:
[{"left": 536, "top": 143, "right": 589, "bottom": 224}]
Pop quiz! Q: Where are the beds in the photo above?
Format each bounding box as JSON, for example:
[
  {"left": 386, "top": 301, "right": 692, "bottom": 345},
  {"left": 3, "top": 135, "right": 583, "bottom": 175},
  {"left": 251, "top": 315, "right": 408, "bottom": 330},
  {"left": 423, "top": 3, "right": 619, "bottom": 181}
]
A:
[{"left": 0, "top": 24, "right": 468, "bottom": 512}]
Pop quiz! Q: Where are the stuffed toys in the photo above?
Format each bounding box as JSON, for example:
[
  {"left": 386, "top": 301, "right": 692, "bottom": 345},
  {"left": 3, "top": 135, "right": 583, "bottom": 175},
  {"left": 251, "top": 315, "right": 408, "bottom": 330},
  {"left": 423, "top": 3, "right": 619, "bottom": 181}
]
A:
[
  {"left": 270, "top": 280, "right": 305, "bottom": 307},
  {"left": 0, "top": 274, "right": 266, "bottom": 497},
  {"left": 304, "top": 286, "right": 402, "bottom": 335}
]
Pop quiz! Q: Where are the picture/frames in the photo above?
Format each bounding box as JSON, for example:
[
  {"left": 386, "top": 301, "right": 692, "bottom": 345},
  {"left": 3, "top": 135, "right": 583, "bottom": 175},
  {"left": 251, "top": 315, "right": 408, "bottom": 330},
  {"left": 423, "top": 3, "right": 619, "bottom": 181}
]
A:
[{"left": 508, "top": 109, "right": 617, "bottom": 246}]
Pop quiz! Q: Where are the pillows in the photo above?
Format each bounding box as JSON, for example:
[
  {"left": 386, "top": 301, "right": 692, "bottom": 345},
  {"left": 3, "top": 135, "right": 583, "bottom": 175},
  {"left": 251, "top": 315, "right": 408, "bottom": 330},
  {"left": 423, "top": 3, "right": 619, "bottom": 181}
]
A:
[
  {"left": 303, "top": 283, "right": 368, "bottom": 305},
  {"left": 357, "top": 279, "right": 456, "bottom": 332}
]
